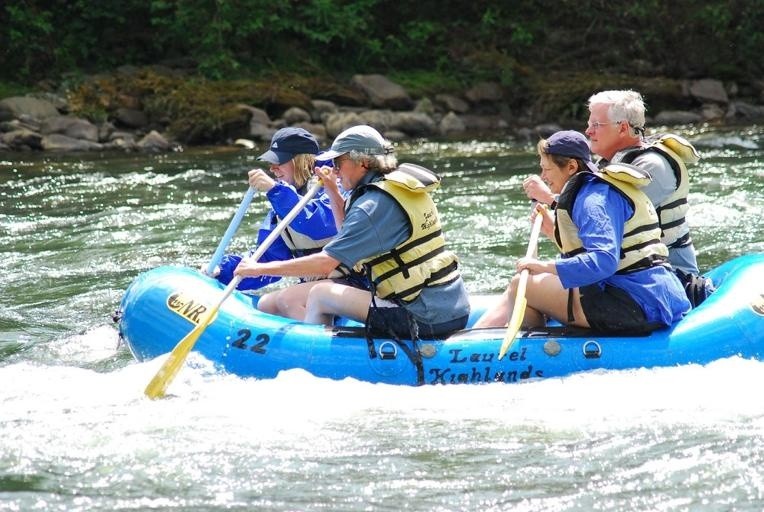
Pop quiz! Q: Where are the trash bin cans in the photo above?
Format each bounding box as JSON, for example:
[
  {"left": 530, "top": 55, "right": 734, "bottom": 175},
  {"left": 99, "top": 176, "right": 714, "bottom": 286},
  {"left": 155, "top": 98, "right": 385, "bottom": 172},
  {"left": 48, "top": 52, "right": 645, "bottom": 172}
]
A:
[{"left": 550, "top": 194, "right": 561, "bottom": 209}]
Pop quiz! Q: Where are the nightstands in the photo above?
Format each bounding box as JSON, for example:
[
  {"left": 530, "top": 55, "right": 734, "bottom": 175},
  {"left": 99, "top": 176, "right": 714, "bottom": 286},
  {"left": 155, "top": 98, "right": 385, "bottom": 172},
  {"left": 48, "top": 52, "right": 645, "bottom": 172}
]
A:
[
  {"left": 499, "top": 203, "right": 547, "bottom": 362},
  {"left": 144, "top": 171, "right": 331, "bottom": 399}
]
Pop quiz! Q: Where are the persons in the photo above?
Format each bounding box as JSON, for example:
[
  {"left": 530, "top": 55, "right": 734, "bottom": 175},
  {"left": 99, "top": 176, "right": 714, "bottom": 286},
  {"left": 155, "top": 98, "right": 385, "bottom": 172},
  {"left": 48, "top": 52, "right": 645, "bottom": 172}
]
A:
[
  {"left": 472, "top": 91, "right": 699, "bottom": 328},
  {"left": 233, "top": 125, "right": 471, "bottom": 339},
  {"left": 507, "top": 128, "right": 691, "bottom": 336},
  {"left": 202, "top": 126, "right": 347, "bottom": 319}
]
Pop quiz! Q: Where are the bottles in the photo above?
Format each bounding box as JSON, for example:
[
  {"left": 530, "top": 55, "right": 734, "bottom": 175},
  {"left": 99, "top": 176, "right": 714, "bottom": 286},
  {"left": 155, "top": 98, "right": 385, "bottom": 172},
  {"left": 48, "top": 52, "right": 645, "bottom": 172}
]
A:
[
  {"left": 543, "top": 129, "right": 601, "bottom": 173},
  {"left": 315, "top": 124, "right": 391, "bottom": 163},
  {"left": 257, "top": 126, "right": 320, "bottom": 166}
]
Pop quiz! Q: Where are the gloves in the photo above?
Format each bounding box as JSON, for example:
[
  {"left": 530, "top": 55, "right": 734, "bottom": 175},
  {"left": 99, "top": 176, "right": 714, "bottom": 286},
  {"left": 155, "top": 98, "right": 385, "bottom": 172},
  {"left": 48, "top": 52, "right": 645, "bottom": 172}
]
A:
[{"left": 119, "top": 253, "right": 764, "bottom": 387}]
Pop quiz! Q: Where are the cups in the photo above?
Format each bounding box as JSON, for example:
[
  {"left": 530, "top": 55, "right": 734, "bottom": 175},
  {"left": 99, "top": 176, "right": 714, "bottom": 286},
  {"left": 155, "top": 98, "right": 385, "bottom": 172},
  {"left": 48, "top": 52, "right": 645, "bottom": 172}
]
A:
[
  {"left": 588, "top": 121, "right": 613, "bottom": 127},
  {"left": 333, "top": 157, "right": 347, "bottom": 171}
]
